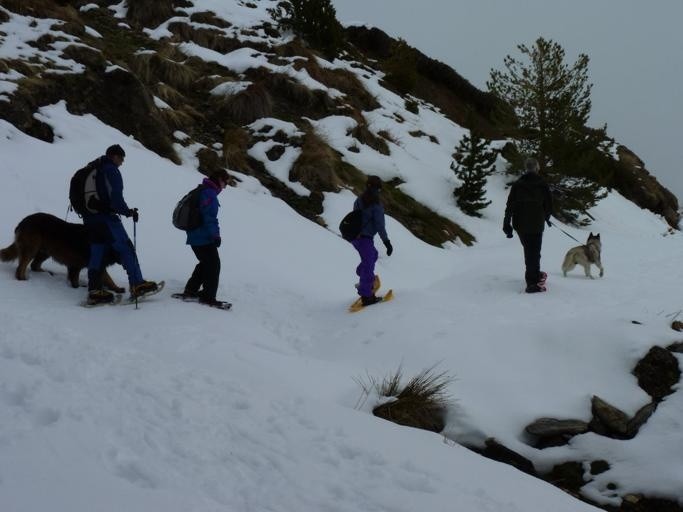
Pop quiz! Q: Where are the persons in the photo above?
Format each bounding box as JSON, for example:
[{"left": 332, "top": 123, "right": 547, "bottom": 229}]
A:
[
  {"left": 502, "top": 157, "right": 555, "bottom": 298},
  {"left": 73, "top": 144, "right": 157, "bottom": 305},
  {"left": 349, "top": 174, "right": 393, "bottom": 308},
  {"left": 181, "top": 168, "right": 229, "bottom": 307}
]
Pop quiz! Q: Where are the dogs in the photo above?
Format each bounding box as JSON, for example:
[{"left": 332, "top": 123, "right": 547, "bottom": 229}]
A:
[
  {"left": 562, "top": 232, "right": 603, "bottom": 279},
  {"left": 0, "top": 213, "right": 125, "bottom": 293}
]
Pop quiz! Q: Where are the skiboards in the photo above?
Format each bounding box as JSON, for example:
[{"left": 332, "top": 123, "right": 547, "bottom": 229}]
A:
[
  {"left": 520, "top": 271, "right": 547, "bottom": 292},
  {"left": 348, "top": 275, "right": 393, "bottom": 313}
]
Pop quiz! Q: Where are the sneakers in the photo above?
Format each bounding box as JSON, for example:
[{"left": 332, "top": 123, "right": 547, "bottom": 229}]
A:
[
  {"left": 129, "top": 280, "right": 158, "bottom": 298},
  {"left": 180, "top": 288, "right": 222, "bottom": 307},
  {"left": 359, "top": 296, "right": 383, "bottom": 307},
  {"left": 525, "top": 271, "right": 546, "bottom": 293},
  {"left": 86, "top": 290, "right": 115, "bottom": 306}
]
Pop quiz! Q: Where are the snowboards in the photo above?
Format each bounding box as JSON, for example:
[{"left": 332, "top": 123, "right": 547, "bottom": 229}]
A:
[{"left": 171, "top": 293, "right": 232, "bottom": 310}]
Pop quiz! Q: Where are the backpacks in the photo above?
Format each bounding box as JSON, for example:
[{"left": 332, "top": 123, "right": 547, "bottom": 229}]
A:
[
  {"left": 339, "top": 210, "right": 364, "bottom": 241},
  {"left": 171, "top": 183, "right": 205, "bottom": 232},
  {"left": 68, "top": 160, "right": 103, "bottom": 219}
]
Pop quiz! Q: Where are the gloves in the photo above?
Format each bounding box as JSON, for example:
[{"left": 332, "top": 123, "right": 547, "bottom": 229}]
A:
[
  {"left": 503, "top": 224, "right": 513, "bottom": 238},
  {"left": 383, "top": 240, "right": 395, "bottom": 256},
  {"left": 545, "top": 214, "right": 552, "bottom": 227},
  {"left": 126, "top": 208, "right": 139, "bottom": 222},
  {"left": 213, "top": 237, "right": 221, "bottom": 248}
]
